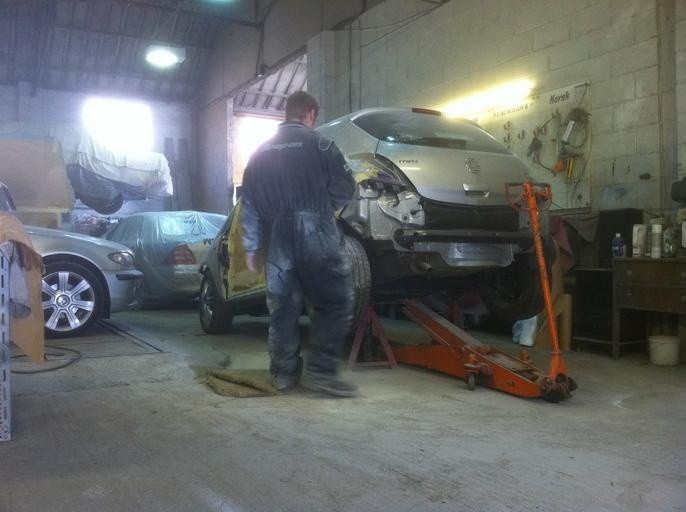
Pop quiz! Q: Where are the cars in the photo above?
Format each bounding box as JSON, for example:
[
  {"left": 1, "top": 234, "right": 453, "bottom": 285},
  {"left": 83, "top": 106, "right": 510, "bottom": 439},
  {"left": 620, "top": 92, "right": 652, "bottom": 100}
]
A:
[
  {"left": 59, "top": 131, "right": 174, "bottom": 214},
  {"left": 97, "top": 209, "right": 224, "bottom": 306},
  {"left": 0, "top": 184, "right": 142, "bottom": 336},
  {"left": 197, "top": 106, "right": 552, "bottom": 335}
]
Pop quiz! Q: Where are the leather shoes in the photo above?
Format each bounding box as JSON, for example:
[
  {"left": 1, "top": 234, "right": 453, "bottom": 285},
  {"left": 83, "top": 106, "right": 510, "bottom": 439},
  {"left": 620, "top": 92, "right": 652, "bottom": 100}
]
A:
[
  {"left": 273, "top": 374, "right": 298, "bottom": 392},
  {"left": 298, "top": 369, "right": 357, "bottom": 397}
]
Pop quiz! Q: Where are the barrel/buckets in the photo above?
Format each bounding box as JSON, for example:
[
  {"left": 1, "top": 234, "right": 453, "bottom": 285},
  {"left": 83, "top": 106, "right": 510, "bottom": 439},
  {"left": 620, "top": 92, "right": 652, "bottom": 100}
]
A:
[{"left": 646, "top": 335, "right": 681, "bottom": 367}]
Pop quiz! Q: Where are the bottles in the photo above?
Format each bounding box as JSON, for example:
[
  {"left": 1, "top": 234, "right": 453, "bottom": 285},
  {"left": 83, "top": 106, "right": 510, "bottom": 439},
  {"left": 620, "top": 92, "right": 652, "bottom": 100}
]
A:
[
  {"left": 612, "top": 232, "right": 625, "bottom": 257},
  {"left": 632, "top": 216, "right": 675, "bottom": 258}
]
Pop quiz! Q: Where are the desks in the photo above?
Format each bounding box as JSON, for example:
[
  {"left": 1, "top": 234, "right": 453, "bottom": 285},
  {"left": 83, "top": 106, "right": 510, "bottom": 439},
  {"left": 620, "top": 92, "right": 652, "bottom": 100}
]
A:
[{"left": 612, "top": 256, "right": 686, "bottom": 361}]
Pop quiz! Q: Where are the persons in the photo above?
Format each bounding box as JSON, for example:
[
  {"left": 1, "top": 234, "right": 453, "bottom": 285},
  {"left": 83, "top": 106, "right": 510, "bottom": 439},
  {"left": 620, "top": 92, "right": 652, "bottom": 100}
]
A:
[{"left": 236, "top": 91, "right": 359, "bottom": 398}]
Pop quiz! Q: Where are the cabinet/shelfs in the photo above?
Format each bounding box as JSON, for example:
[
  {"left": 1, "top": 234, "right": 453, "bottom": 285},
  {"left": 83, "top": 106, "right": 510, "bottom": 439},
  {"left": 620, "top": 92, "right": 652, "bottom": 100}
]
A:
[{"left": 571, "top": 267, "right": 651, "bottom": 354}]
